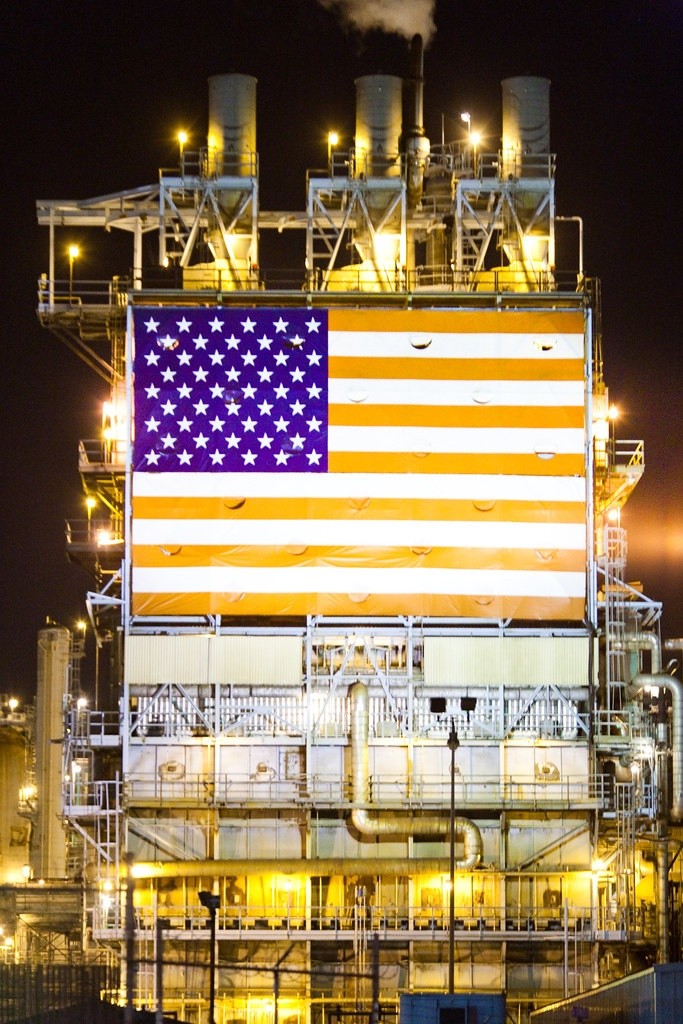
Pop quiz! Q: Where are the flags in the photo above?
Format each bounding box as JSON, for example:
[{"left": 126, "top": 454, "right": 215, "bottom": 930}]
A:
[{"left": 125, "top": 302, "right": 588, "bottom": 620}]
